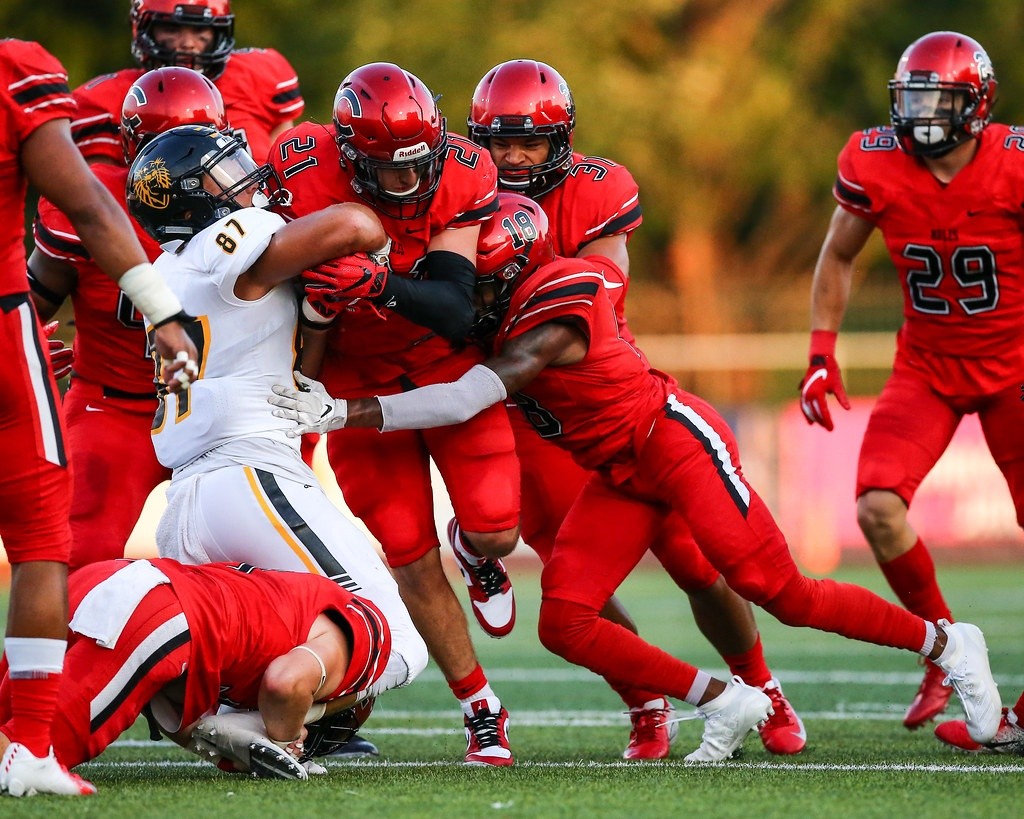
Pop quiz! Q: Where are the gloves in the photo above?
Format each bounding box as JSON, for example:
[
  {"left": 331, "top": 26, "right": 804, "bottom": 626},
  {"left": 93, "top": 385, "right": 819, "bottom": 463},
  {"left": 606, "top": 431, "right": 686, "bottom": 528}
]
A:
[
  {"left": 300, "top": 253, "right": 388, "bottom": 299},
  {"left": 800, "top": 329, "right": 850, "bottom": 432},
  {"left": 296, "top": 280, "right": 343, "bottom": 327},
  {"left": 267, "top": 370, "right": 347, "bottom": 438},
  {"left": 43, "top": 320, "right": 75, "bottom": 380}
]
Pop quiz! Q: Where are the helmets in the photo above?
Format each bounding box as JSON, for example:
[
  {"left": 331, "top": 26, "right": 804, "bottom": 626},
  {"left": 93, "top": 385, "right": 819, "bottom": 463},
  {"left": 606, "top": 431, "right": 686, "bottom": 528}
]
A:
[
  {"left": 462, "top": 194, "right": 555, "bottom": 340},
  {"left": 333, "top": 62, "right": 448, "bottom": 220},
  {"left": 125, "top": 124, "right": 282, "bottom": 253},
  {"left": 129, "top": 0, "right": 234, "bottom": 81},
  {"left": 886, "top": 30, "right": 997, "bottom": 158},
  {"left": 120, "top": 69, "right": 228, "bottom": 166},
  {"left": 466, "top": 59, "right": 577, "bottom": 199}
]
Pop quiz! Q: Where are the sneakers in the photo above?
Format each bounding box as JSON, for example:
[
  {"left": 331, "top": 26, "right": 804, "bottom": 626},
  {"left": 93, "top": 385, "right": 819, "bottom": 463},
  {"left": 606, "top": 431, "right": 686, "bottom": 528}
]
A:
[
  {"left": 459, "top": 696, "right": 514, "bottom": 770},
  {"left": 447, "top": 517, "right": 516, "bottom": 639},
  {"left": 0, "top": 742, "right": 98, "bottom": 798},
  {"left": 932, "top": 619, "right": 1002, "bottom": 743},
  {"left": 931, "top": 707, "right": 1024, "bottom": 755},
  {"left": 683, "top": 675, "right": 775, "bottom": 763},
  {"left": 901, "top": 655, "right": 954, "bottom": 733},
  {"left": 623, "top": 695, "right": 680, "bottom": 761},
  {"left": 758, "top": 684, "right": 807, "bottom": 756},
  {"left": 303, "top": 728, "right": 379, "bottom": 759},
  {"left": 191, "top": 713, "right": 310, "bottom": 782}
]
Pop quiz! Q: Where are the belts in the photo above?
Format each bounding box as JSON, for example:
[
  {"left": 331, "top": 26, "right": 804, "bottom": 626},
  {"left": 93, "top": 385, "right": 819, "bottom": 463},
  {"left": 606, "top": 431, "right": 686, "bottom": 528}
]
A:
[{"left": 104, "top": 384, "right": 158, "bottom": 400}]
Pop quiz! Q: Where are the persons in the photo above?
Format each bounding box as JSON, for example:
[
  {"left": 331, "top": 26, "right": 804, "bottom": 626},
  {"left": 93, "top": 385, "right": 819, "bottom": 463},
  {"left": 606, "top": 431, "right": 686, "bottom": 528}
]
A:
[
  {"left": 799, "top": 31, "right": 1024, "bottom": 748},
  {"left": 0, "top": 1, "right": 1001, "bottom": 796}
]
